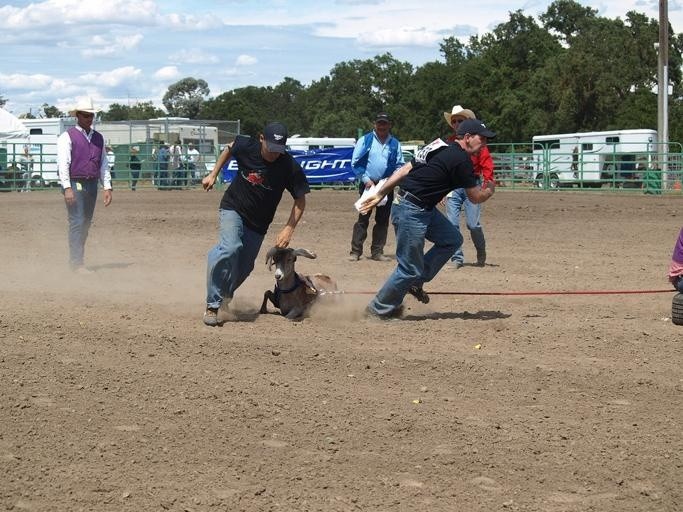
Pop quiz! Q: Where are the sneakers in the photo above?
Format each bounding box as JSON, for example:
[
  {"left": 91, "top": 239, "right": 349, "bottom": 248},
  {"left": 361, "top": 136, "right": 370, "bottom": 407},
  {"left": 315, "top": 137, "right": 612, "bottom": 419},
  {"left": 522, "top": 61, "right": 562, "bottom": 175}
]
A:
[
  {"left": 448, "top": 260, "right": 462, "bottom": 268},
  {"left": 364, "top": 305, "right": 404, "bottom": 320},
  {"left": 347, "top": 254, "right": 359, "bottom": 261},
  {"left": 203, "top": 304, "right": 218, "bottom": 325},
  {"left": 409, "top": 284, "right": 429, "bottom": 304},
  {"left": 217, "top": 302, "right": 229, "bottom": 321},
  {"left": 477, "top": 250, "right": 486, "bottom": 264},
  {"left": 372, "top": 253, "right": 392, "bottom": 261}
]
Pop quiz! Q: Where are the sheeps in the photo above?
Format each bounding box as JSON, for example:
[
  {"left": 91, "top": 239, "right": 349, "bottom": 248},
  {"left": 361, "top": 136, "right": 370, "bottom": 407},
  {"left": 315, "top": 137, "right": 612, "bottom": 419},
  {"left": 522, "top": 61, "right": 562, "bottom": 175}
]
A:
[{"left": 259, "top": 244, "right": 337, "bottom": 322}]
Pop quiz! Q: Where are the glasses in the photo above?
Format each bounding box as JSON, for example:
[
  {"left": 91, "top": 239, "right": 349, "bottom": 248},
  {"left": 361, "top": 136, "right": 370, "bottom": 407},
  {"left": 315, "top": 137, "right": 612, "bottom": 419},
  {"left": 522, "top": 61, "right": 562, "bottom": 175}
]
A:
[
  {"left": 80, "top": 113, "right": 94, "bottom": 118},
  {"left": 451, "top": 119, "right": 463, "bottom": 123}
]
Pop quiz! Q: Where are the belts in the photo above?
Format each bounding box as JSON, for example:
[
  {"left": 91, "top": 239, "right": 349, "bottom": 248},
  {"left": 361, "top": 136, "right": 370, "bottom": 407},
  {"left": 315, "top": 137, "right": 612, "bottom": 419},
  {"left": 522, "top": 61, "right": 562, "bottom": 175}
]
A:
[{"left": 398, "top": 190, "right": 433, "bottom": 210}]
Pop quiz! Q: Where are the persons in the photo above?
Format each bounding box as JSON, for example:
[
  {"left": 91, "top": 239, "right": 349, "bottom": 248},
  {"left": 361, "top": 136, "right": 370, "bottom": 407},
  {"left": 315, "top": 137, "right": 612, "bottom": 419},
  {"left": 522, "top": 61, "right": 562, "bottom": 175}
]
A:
[
  {"left": 16, "top": 147, "right": 35, "bottom": 193},
  {"left": 444, "top": 106, "right": 496, "bottom": 270},
  {"left": 359, "top": 118, "right": 496, "bottom": 319},
  {"left": 56, "top": 99, "right": 112, "bottom": 277},
  {"left": 105, "top": 141, "right": 201, "bottom": 192},
  {"left": 347, "top": 114, "right": 407, "bottom": 261},
  {"left": 201, "top": 122, "right": 310, "bottom": 325},
  {"left": 667, "top": 228, "right": 683, "bottom": 297}
]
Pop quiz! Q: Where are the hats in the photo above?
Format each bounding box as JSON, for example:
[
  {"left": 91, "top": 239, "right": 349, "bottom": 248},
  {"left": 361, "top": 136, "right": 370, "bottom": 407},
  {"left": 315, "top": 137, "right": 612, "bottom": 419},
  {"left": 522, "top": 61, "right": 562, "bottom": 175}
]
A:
[
  {"left": 67, "top": 97, "right": 103, "bottom": 116},
  {"left": 456, "top": 118, "right": 496, "bottom": 140},
  {"left": 376, "top": 111, "right": 390, "bottom": 123},
  {"left": 444, "top": 105, "right": 477, "bottom": 128},
  {"left": 264, "top": 122, "right": 288, "bottom": 155}
]
